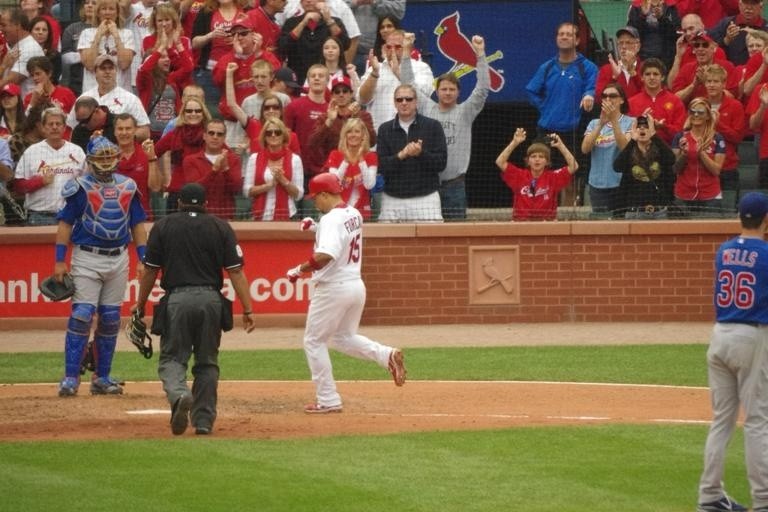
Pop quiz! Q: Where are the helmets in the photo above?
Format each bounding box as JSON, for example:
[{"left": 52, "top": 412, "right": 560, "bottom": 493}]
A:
[{"left": 86, "top": 137, "right": 120, "bottom": 176}]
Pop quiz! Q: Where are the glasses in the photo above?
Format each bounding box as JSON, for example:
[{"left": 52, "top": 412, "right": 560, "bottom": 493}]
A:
[
  {"left": 77, "top": 112, "right": 93, "bottom": 124},
  {"left": 184, "top": 109, "right": 225, "bottom": 138},
  {"left": 264, "top": 105, "right": 280, "bottom": 110},
  {"left": 232, "top": 31, "right": 248, "bottom": 37},
  {"left": 335, "top": 89, "right": 348, "bottom": 94},
  {"left": 265, "top": 130, "right": 281, "bottom": 136},
  {"left": 688, "top": 108, "right": 705, "bottom": 115},
  {"left": 396, "top": 98, "right": 413, "bottom": 102},
  {"left": 694, "top": 43, "right": 710, "bottom": 48},
  {"left": 601, "top": 93, "right": 620, "bottom": 98},
  {"left": 386, "top": 45, "right": 401, "bottom": 49}
]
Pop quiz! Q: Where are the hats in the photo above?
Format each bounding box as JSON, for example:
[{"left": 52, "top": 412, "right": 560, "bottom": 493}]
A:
[
  {"left": 230, "top": 18, "right": 252, "bottom": 31},
  {"left": 180, "top": 183, "right": 205, "bottom": 205},
  {"left": 332, "top": 76, "right": 350, "bottom": 90},
  {"left": 303, "top": 173, "right": 342, "bottom": 199},
  {"left": 0, "top": 83, "right": 20, "bottom": 96},
  {"left": 616, "top": 26, "right": 639, "bottom": 38},
  {"left": 738, "top": 193, "right": 768, "bottom": 217},
  {"left": 94, "top": 54, "right": 115, "bottom": 67}
]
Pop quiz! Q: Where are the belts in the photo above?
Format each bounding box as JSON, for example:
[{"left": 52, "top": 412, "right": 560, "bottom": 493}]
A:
[{"left": 80, "top": 245, "right": 126, "bottom": 256}]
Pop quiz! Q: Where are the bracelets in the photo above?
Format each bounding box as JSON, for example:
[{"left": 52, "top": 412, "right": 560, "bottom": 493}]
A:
[
  {"left": 137, "top": 245, "right": 146, "bottom": 263},
  {"left": 243, "top": 311, "right": 252, "bottom": 315},
  {"left": 55, "top": 243, "right": 67, "bottom": 262}
]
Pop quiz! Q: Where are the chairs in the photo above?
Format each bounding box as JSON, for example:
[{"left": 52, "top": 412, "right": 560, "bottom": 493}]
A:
[
  {"left": 146, "top": 96, "right": 323, "bottom": 225},
  {"left": 704, "top": 138, "right": 767, "bottom": 213}
]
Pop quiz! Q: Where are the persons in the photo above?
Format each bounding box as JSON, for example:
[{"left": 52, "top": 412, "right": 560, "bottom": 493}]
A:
[
  {"left": 52, "top": 136, "right": 148, "bottom": 396},
  {"left": 697, "top": 191, "right": 768, "bottom": 511},
  {"left": 286, "top": 173, "right": 407, "bottom": 413},
  {"left": 129, "top": 182, "right": 257, "bottom": 436}
]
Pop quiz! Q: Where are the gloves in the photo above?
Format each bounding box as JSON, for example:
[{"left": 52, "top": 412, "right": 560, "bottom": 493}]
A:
[
  {"left": 301, "top": 217, "right": 317, "bottom": 232},
  {"left": 287, "top": 265, "right": 303, "bottom": 283}
]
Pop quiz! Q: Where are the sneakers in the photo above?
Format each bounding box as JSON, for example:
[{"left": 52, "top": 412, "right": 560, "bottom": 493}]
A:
[
  {"left": 304, "top": 403, "right": 343, "bottom": 413},
  {"left": 698, "top": 495, "right": 747, "bottom": 512},
  {"left": 389, "top": 349, "right": 406, "bottom": 386},
  {"left": 59, "top": 374, "right": 122, "bottom": 394},
  {"left": 171, "top": 392, "right": 192, "bottom": 435}
]
